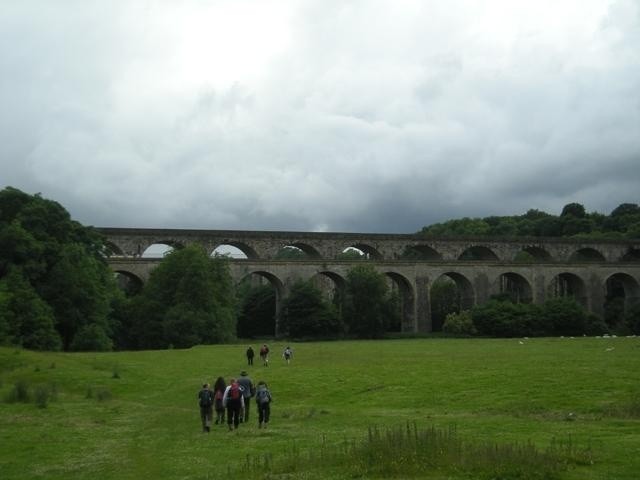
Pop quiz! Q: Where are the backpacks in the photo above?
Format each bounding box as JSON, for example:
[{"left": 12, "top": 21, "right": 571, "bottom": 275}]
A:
[
  {"left": 215, "top": 390, "right": 223, "bottom": 400},
  {"left": 200, "top": 390, "right": 211, "bottom": 407},
  {"left": 228, "top": 382, "right": 241, "bottom": 402},
  {"left": 258, "top": 391, "right": 270, "bottom": 406}
]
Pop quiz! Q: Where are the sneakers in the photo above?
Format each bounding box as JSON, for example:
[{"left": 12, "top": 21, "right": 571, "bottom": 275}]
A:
[{"left": 203, "top": 419, "right": 268, "bottom": 432}]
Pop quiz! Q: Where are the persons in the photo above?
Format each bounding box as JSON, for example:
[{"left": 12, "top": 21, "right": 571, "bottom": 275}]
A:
[
  {"left": 214, "top": 376, "right": 227, "bottom": 425},
  {"left": 222, "top": 378, "right": 245, "bottom": 432},
  {"left": 284, "top": 346, "right": 294, "bottom": 364},
  {"left": 197, "top": 383, "right": 215, "bottom": 433},
  {"left": 260, "top": 344, "right": 269, "bottom": 366},
  {"left": 254, "top": 380, "right": 273, "bottom": 429},
  {"left": 247, "top": 347, "right": 254, "bottom": 366},
  {"left": 235, "top": 371, "right": 256, "bottom": 425}
]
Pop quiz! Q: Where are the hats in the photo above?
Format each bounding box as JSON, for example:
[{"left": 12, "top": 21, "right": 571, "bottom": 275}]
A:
[{"left": 240, "top": 370, "right": 248, "bottom": 375}]
